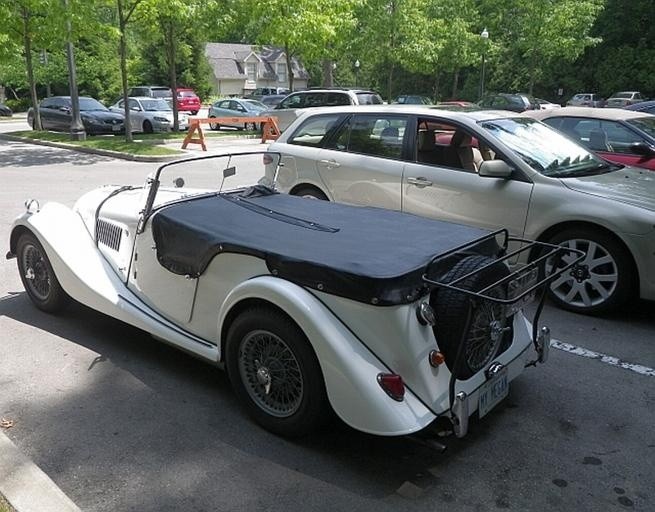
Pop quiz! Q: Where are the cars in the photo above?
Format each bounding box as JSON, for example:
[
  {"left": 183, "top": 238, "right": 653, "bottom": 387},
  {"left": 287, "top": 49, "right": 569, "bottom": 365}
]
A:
[
  {"left": 534, "top": 98, "right": 561, "bottom": 111},
  {"left": 177, "top": 88, "right": 201, "bottom": 115},
  {"left": 208, "top": 98, "right": 270, "bottom": 131},
  {"left": 27, "top": 95, "right": 126, "bottom": 137},
  {"left": 576, "top": 100, "right": 655, "bottom": 144},
  {"left": 422, "top": 101, "right": 481, "bottom": 147},
  {"left": 391, "top": 95, "right": 433, "bottom": 105},
  {"left": 258, "top": 94, "right": 286, "bottom": 105},
  {"left": 6, "top": 151, "right": 586, "bottom": 455},
  {"left": 108, "top": 96, "right": 190, "bottom": 134},
  {"left": 519, "top": 106, "right": 655, "bottom": 171},
  {"left": 244, "top": 86, "right": 290, "bottom": 105},
  {"left": 0, "top": 103, "right": 13, "bottom": 117},
  {"left": 603, "top": 91, "right": 650, "bottom": 108}
]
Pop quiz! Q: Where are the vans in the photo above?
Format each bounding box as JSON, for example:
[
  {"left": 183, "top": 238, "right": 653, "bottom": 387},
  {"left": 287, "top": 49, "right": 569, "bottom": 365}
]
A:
[
  {"left": 566, "top": 93, "right": 603, "bottom": 108},
  {"left": 255, "top": 87, "right": 383, "bottom": 139},
  {"left": 127, "top": 85, "right": 173, "bottom": 104}
]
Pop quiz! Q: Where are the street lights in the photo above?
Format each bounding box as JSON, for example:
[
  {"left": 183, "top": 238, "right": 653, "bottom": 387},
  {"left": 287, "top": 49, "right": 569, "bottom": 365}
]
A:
[
  {"left": 479, "top": 28, "right": 489, "bottom": 98},
  {"left": 354, "top": 59, "right": 360, "bottom": 90},
  {"left": 332, "top": 60, "right": 337, "bottom": 85}
]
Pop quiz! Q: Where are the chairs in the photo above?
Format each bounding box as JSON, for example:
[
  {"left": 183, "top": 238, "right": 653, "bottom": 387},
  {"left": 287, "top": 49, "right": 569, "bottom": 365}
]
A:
[
  {"left": 417, "top": 131, "right": 445, "bottom": 165},
  {"left": 479, "top": 139, "right": 496, "bottom": 160},
  {"left": 450, "top": 129, "right": 484, "bottom": 173},
  {"left": 589, "top": 128, "right": 615, "bottom": 153}
]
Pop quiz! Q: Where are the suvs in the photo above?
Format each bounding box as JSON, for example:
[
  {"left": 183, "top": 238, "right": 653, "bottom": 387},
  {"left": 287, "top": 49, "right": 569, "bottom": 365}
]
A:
[
  {"left": 258, "top": 105, "right": 655, "bottom": 315},
  {"left": 473, "top": 90, "right": 540, "bottom": 113}
]
